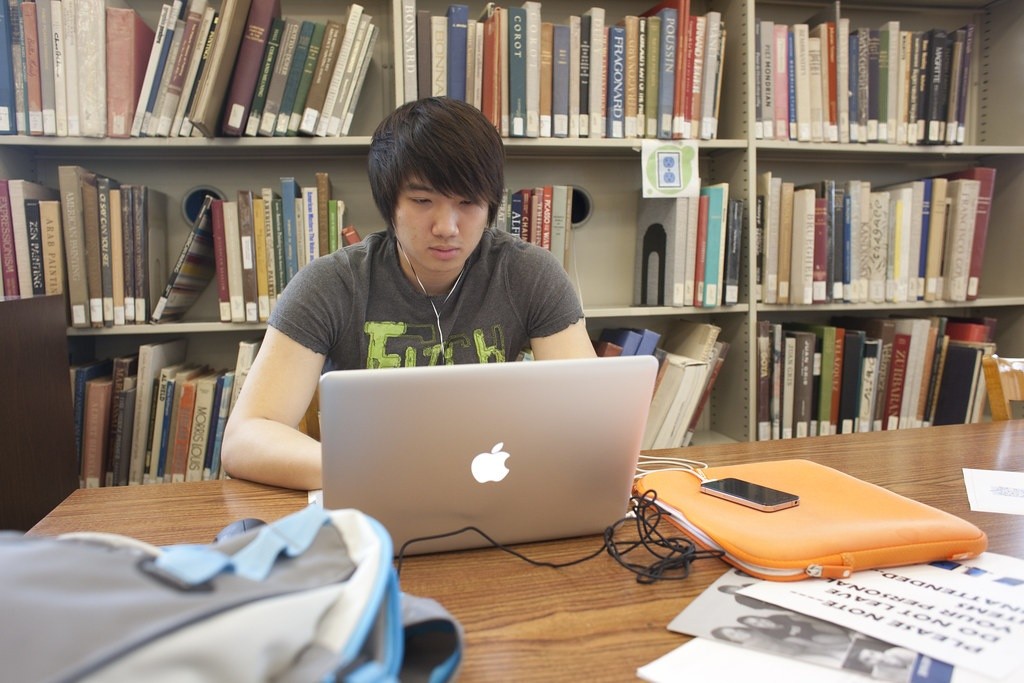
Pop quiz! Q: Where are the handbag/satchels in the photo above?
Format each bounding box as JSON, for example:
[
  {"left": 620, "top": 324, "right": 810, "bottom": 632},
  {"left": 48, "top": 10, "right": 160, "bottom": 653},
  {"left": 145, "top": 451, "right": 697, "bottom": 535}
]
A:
[{"left": 637, "top": 459, "right": 988, "bottom": 581}]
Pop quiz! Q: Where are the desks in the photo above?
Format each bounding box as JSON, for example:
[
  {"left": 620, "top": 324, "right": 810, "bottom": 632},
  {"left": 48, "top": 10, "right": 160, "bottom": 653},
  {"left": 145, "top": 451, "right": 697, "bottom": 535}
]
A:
[
  {"left": 22, "top": 420, "right": 1024, "bottom": 683},
  {"left": 0, "top": 294, "right": 79, "bottom": 531}
]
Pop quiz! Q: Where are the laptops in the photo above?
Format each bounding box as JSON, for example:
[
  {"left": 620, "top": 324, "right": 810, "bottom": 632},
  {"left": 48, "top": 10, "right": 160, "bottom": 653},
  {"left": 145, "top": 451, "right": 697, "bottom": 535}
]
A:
[{"left": 317, "top": 353, "right": 658, "bottom": 558}]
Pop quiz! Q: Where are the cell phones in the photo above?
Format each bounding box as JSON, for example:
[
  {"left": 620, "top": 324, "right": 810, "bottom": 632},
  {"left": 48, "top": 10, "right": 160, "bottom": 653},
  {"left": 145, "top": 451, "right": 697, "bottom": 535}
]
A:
[{"left": 700, "top": 478, "right": 800, "bottom": 513}]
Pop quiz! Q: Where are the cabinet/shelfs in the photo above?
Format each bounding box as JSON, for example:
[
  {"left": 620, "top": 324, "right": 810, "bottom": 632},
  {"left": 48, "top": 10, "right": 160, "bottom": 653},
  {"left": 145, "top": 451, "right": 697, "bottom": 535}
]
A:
[{"left": 0, "top": 0, "right": 1024, "bottom": 439}]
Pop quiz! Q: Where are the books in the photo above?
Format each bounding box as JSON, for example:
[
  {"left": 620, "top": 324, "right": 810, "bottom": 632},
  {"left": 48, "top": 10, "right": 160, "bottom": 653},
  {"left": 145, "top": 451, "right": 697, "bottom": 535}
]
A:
[
  {"left": 0, "top": 163, "right": 999, "bottom": 305},
  {"left": 595, "top": 320, "right": 732, "bottom": 451},
  {"left": 0, "top": 0, "right": 975, "bottom": 147},
  {"left": 758, "top": 317, "right": 998, "bottom": 442},
  {"left": 68, "top": 337, "right": 262, "bottom": 484}
]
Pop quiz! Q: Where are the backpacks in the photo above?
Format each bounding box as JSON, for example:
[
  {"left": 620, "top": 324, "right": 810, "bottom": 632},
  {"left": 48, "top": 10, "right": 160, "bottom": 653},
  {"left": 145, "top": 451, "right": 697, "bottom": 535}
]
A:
[{"left": 0, "top": 508, "right": 464, "bottom": 683}]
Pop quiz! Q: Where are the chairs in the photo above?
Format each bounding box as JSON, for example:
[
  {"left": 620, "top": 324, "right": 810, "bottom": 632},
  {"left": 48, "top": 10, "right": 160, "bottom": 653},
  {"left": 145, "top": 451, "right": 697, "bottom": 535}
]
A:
[{"left": 982, "top": 354, "right": 1024, "bottom": 422}]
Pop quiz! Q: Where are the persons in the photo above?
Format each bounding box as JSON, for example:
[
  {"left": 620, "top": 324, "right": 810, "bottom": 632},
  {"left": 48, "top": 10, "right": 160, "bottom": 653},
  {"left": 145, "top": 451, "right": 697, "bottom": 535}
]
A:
[
  {"left": 218, "top": 99, "right": 602, "bottom": 485},
  {"left": 711, "top": 570, "right": 918, "bottom": 682}
]
